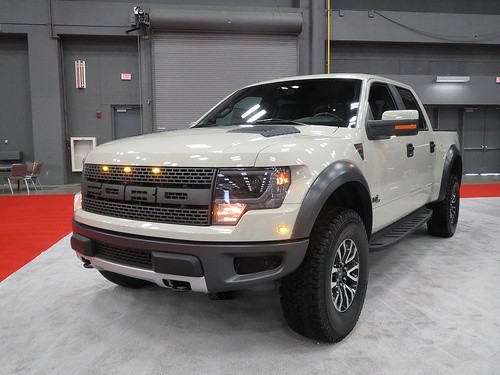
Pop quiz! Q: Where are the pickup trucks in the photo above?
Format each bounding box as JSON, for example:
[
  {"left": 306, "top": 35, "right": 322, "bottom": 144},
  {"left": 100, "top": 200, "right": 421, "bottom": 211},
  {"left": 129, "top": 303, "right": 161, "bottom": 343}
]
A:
[{"left": 70, "top": 69, "right": 466, "bottom": 341}]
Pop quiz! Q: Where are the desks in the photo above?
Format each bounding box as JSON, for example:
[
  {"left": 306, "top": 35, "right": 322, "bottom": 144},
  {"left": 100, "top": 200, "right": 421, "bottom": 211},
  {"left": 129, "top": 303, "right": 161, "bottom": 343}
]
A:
[{"left": 0, "top": 166, "right": 20, "bottom": 193}]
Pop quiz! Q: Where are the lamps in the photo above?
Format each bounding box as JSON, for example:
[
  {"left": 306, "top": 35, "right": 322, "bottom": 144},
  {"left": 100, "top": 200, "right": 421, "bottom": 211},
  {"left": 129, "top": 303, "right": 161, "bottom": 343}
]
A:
[{"left": 435, "top": 75, "right": 470, "bottom": 83}]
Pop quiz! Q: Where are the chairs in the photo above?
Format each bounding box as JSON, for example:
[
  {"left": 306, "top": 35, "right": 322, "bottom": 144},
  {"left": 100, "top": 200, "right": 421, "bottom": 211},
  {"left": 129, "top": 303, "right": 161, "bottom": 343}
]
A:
[
  {"left": 23, "top": 161, "right": 35, "bottom": 191},
  {"left": 3, "top": 164, "right": 30, "bottom": 195},
  {"left": 19, "top": 161, "right": 43, "bottom": 191}
]
